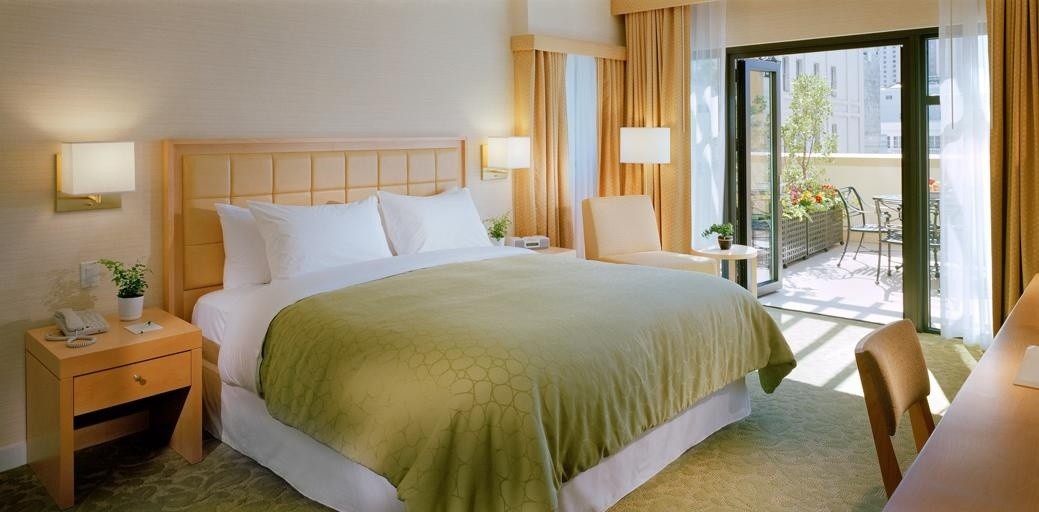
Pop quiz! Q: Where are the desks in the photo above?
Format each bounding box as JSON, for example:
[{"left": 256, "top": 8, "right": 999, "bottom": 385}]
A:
[
  {"left": 881, "top": 268, "right": 1037, "bottom": 512},
  {"left": 695, "top": 244, "right": 760, "bottom": 295}
]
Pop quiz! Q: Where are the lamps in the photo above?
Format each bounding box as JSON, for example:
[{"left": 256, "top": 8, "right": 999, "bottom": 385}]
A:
[
  {"left": 478, "top": 135, "right": 532, "bottom": 182},
  {"left": 49, "top": 135, "right": 134, "bottom": 215},
  {"left": 619, "top": 126, "right": 672, "bottom": 195}
]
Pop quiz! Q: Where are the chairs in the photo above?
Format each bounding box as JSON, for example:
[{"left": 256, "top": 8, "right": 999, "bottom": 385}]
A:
[
  {"left": 854, "top": 316, "right": 935, "bottom": 496},
  {"left": 578, "top": 192, "right": 713, "bottom": 273},
  {"left": 832, "top": 184, "right": 940, "bottom": 291}
]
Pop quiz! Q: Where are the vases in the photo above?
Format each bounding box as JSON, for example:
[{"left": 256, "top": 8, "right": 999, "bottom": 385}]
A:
[{"left": 776, "top": 206, "right": 844, "bottom": 268}]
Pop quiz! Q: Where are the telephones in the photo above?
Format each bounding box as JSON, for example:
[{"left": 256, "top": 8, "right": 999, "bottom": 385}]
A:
[{"left": 53, "top": 308, "right": 110, "bottom": 337}]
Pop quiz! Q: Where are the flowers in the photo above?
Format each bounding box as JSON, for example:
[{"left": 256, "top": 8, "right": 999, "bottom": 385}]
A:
[{"left": 779, "top": 172, "right": 839, "bottom": 215}]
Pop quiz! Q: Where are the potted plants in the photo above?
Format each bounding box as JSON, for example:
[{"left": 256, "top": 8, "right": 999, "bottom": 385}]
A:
[
  {"left": 483, "top": 211, "right": 513, "bottom": 246},
  {"left": 97, "top": 253, "right": 152, "bottom": 321},
  {"left": 704, "top": 221, "right": 736, "bottom": 248}
]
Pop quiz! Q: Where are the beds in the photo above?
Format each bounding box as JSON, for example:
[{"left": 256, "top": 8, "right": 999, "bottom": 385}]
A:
[{"left": 158, "top": 133, "right": 798, "bottom": 512}]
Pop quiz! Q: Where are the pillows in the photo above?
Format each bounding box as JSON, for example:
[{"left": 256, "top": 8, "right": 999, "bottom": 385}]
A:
[
  {"left": 212, "top": 202, "right": 268, "bottom": 292},
  {"left": 372, "top": 183, "right": 495, "bottom": 255},
  {"left": 244, "top": 199, "right": 394, "bottom": 281}
]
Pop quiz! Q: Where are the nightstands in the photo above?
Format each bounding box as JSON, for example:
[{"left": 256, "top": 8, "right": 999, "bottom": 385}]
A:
[
  {"left": 23, "top": 307, "right": 206, "bottom": 509},
  {"left": 529, "top": 245, "right": 578, "bottom": 259}
]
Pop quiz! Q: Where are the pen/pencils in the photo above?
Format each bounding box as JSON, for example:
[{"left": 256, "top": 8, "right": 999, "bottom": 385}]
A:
[{"left": 141, "top": 321, "right": 151, "bottom": 334}]
